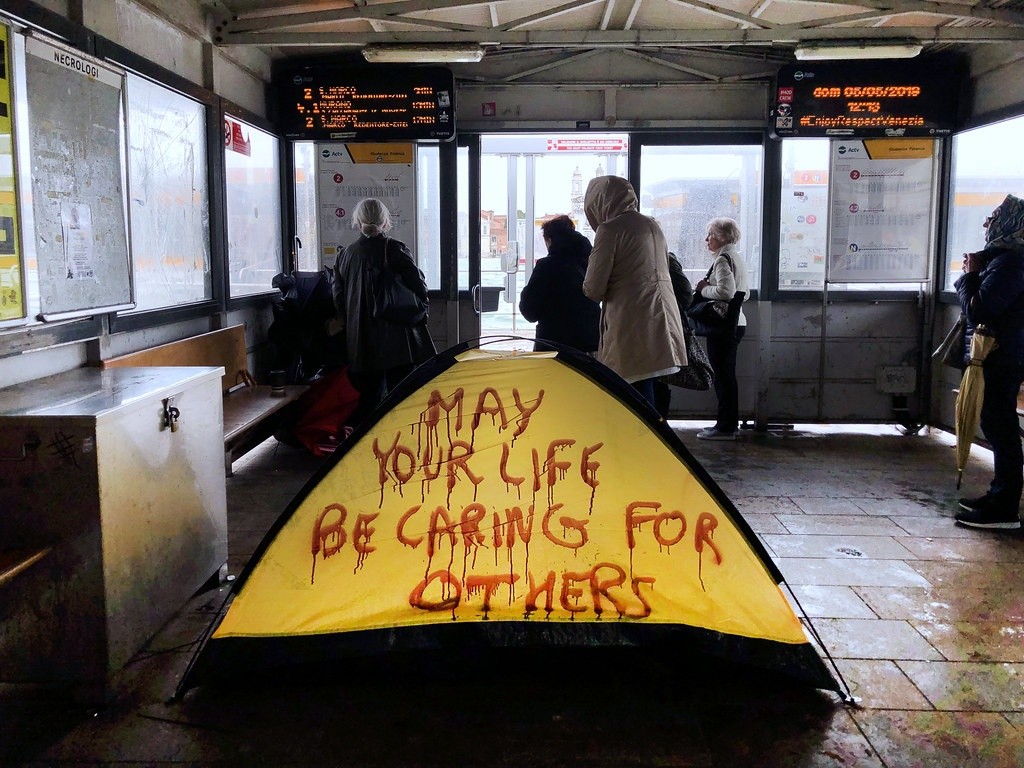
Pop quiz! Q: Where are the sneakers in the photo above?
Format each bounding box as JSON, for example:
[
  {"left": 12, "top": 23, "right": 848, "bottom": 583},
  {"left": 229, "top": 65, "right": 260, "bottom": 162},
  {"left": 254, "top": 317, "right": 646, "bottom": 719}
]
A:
[
  {"left": 957, "top": 491, "right": 992, "bottom": 513},
  {"left": 952, "top": 508, "right": 1021, "bottom": 531},
  {"left": 703, "top": 424, "right": 740, "bottom": 435},
  {"left": 697, "top": 429, "right": 737, "bottom": 441}
]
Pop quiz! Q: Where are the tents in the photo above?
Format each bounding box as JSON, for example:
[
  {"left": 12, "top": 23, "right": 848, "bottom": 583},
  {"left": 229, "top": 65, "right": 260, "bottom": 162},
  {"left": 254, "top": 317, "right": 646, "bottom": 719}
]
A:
[{"left": 163, "top": 334, "right": 860, "bottom": 710}]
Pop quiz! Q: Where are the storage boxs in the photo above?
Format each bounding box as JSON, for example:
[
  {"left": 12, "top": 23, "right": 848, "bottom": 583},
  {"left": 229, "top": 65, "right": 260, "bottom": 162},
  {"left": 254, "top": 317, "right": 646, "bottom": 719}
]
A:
[{"left": 1, "top": 366, "right": 229, "bottom": 679}]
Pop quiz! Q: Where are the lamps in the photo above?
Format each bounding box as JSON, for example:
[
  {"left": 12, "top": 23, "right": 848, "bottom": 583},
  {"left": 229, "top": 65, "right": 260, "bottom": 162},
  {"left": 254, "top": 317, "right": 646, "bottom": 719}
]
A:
[
  {"left": 794, "top": 38, "right": 926, "bottom": 62},
  {"left": 363, "top": 44, "right": 487, "bottom": 64}
]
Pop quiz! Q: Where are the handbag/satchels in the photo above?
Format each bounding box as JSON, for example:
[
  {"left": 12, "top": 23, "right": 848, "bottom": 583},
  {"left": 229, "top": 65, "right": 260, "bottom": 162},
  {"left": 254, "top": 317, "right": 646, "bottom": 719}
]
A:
[
  {"left": 366, "top": 236, "right": 432, "bottom": 325},
  {"left": 662, "top": 311, "right": 713, "bottom": 392},
  {"left": 687, "top": 253, "right": 747, "bottom": 341},
  {"left": 930, "top": 307, "right": 966, "bottom": 373}
]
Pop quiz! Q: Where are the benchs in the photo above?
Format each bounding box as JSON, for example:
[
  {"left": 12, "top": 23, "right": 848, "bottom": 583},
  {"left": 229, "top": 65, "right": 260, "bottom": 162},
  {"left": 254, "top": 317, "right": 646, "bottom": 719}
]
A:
[{"left": 102, "top": 323, "right": 311, "bottom": 477}]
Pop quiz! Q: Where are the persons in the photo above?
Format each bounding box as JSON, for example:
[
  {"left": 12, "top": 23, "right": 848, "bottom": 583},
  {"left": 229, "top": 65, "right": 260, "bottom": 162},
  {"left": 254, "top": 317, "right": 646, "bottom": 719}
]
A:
[
  {"left": 519, "top": 215, "right": 604, "bottom": 355},
  {"left": 582, "top": 175, "right": 689, "bottom": 410},
  {"left": 695, "top": 216, "right": 751, "bottom": 441},
  {"left": 955, "top": 194, "right": 1024, "bottom": 529},
  {"left": 331, "top": 198, "right": 438, "bottom": 395}
]
